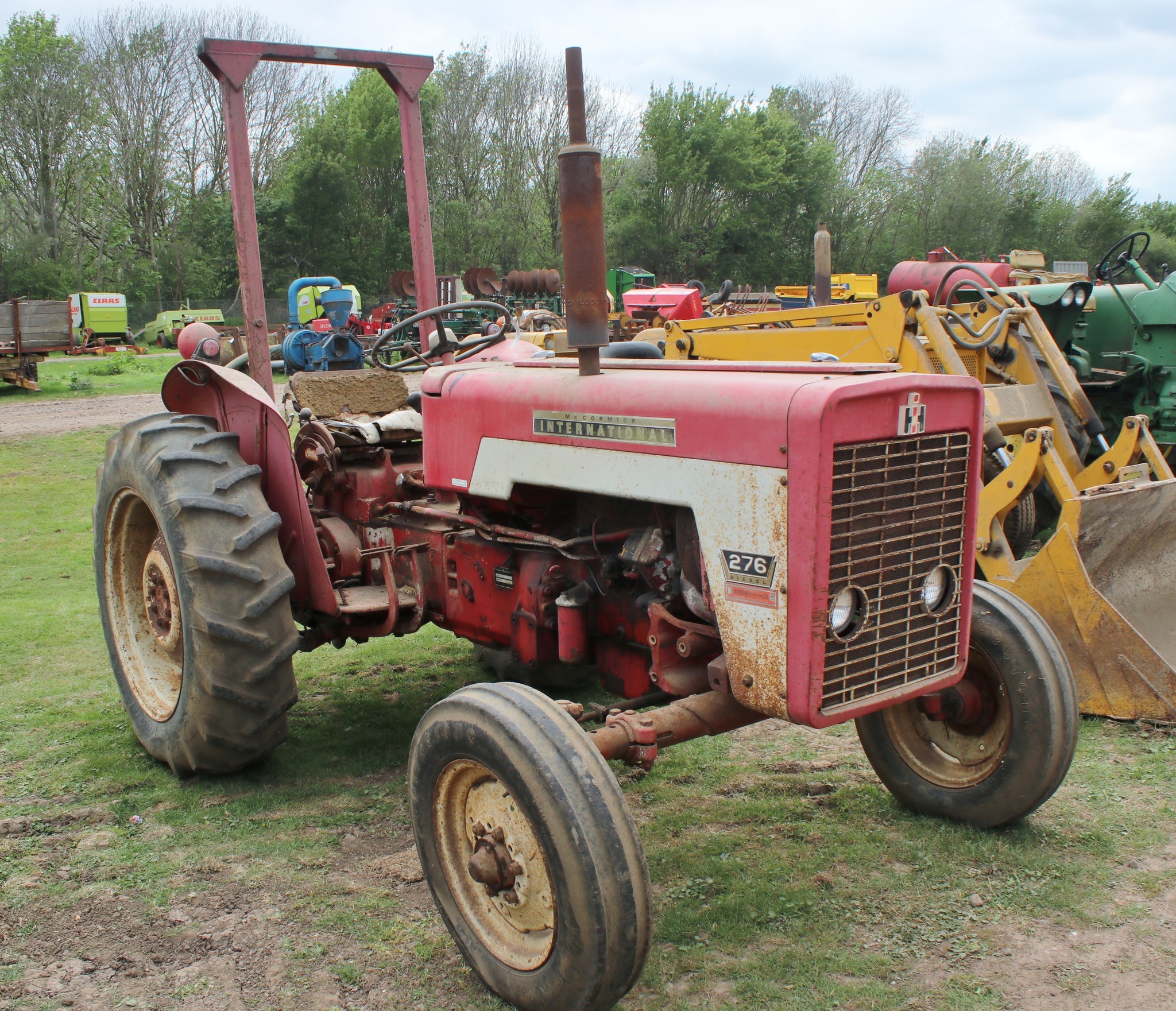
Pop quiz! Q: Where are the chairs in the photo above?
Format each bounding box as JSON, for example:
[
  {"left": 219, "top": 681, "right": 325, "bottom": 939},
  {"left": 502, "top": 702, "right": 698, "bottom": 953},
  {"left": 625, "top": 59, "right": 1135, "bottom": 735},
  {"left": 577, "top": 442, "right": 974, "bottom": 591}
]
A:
[{"left": 282, "top": 368, "right": 422, "bottom": 446}]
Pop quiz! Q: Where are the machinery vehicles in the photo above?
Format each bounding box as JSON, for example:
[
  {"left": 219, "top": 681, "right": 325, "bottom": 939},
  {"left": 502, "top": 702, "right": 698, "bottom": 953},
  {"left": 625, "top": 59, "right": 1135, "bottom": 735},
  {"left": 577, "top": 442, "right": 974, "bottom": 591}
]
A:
[
  {"left": 90, "top": 34, "right": 1087, "bottom": 1011},
  {"left": 492, "top": 217, "right": 1175, "bottom": 731}
]
